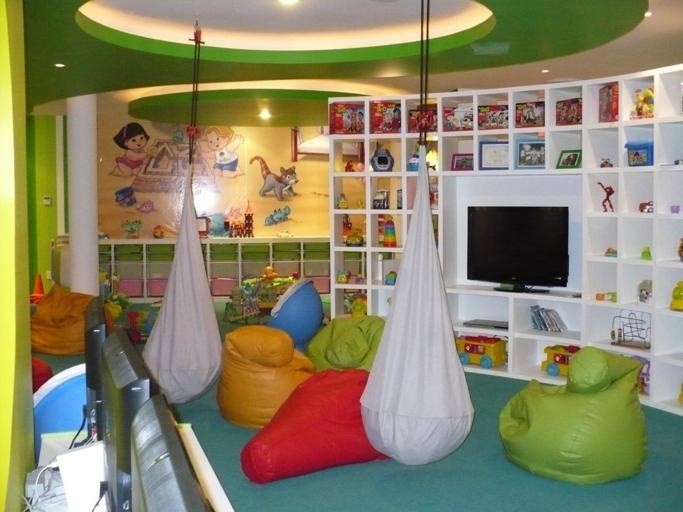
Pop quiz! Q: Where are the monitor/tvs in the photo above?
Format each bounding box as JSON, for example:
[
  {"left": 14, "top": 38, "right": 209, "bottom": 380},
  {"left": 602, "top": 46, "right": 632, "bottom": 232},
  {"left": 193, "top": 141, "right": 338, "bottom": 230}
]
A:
[
  {"left": 467, "top": 206, "right": 569, "bottom": 294},
  {"left": 101, "top": 327, "right": 150, "bottom": 512},
  {"left": 83, "top": 295, "right": 108, "bottom": 443},
  {"left": 130, "top": 393, "right": 206, "bottom": 512}
]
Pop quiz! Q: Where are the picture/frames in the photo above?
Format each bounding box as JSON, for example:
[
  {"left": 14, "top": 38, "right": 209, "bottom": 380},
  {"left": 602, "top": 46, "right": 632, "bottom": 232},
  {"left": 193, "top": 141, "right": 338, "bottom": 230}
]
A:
[
  {"left": 514, "top": 137, "right": 545, "bottom": 169},
  {"left": 450, "top": 154, "right": 474, "bottom": 171},
  {"left": 479, "top": 141, "right": 508, "bottom": 170},
  {"left": 555, "top": 149, "right": 582, "bottom": 169}
]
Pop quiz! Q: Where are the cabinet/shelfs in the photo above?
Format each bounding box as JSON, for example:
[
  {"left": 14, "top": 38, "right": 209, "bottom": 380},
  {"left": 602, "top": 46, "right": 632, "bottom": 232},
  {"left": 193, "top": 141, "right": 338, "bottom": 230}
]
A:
[{"left": 326, "top": 61, "right": 683, "bottom": 420}]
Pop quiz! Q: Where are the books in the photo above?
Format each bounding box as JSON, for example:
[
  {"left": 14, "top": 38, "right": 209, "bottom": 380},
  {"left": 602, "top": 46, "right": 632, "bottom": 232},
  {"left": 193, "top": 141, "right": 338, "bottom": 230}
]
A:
[{"left": 528, "top": 304, "right": 567, "bottom": 333}]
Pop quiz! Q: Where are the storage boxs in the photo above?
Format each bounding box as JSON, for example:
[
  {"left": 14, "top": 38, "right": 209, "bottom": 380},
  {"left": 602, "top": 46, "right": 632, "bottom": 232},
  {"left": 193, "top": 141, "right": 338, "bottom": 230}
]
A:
[{"left": 624, "top": 140, "right": 653, "bottom": 167}]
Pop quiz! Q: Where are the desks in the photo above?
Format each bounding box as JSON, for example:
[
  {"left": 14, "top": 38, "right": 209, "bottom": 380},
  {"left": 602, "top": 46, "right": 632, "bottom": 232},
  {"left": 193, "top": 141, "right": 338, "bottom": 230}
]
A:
[{"left": 35, "top": 422, "right": 236, "bottom": 510}]
